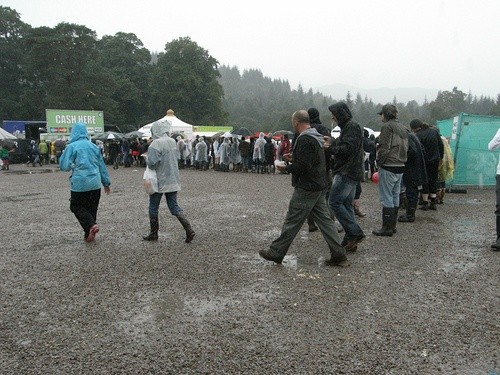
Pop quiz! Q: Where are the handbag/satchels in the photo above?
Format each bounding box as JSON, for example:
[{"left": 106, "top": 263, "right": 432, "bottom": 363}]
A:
[{"left": 143, "top": 165, "right": 158, "bottom": 195}]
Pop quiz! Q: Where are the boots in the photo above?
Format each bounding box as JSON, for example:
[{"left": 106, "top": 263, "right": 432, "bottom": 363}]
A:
[
  {"left": 398, "top": 202, "right": 417, "bottom": 223},
  {"left": 176, "top": 212, "right": 195, "bottom": 243},
  {"left": 428, "top": 199, "right": 437, "bottom": 210},
  {"left": 373, "top": 206, "right": 398, "bottom": 237},
  {"left": 142, "top": 217, "right": 158, "bottom": 241},
  {"left": 419, "top": 201, "right": 429, "bottom": 211},
  {"left": 491, "top": 216, "right": 500, "bottom": 249}
]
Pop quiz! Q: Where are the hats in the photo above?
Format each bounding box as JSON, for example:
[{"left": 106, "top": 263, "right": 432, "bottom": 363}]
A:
[{"left": 377, "top": 105, "right": 397, "bottom": 120}]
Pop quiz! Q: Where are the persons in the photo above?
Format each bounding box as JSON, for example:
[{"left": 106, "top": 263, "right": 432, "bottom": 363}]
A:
[
  {"left": 0, "top": 128, "right": 380, "bottom": 181},
  {"left": 397, "top": 116, "right": 448, "bottom": 221},
  {"left": 258, "top": 101, "right": 368, "bottom": 266},
  {"left": 488, "top": 127, "right": 500, "bottom": 250},
  {"left": 60, "top": 123, "right": 112, "bottom": 242},
  {"left": 142, "top": 121, "right": 196, "bottom": 243},
  {"left": 372, "top": 104, "right": 409, "bottom": 236}
]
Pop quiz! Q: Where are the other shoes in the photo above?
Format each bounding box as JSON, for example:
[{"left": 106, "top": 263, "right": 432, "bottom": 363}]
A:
[
  {"left": 309, "top": 224, "right": 318, "bottom": 232},
  {"left": 436, "top": 189, "right": 445, "bottom": 204},
  {"left": 179, "top": 163, "right": 275, "bottom": 175},
  {"left": 342, "top": 233, "right": 366, "bottom": 249},
  {"left": 325, "top": 257, "right": 348, "bottom": 267},
  {"left": 87, "top": 224, "right": 99, "bottom": 242},
  {"left": 259, "top": 248, "right": 283, "bottom": 265},
  {"left": 354, "top": 209, "right": 366, "bottom": 217}
]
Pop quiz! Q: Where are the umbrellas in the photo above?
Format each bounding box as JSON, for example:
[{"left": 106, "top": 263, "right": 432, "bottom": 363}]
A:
[
  {"left": 130, "top": 130, "right": 144, "bottom": 138},
  {"left": 171, "top": 130, "right": 187, "bottom": 140},
  {"left": 272, "top": 129, "right": 295, "bottom": 135},
  {"left": 0, "top": 127, "right": 18, "bottom": 140},
  {"left": 94, "top": 129, "right": 126, "bottom": 140},
  {"left": 212, "top": 126, "right": 256, "bottom": 139}
]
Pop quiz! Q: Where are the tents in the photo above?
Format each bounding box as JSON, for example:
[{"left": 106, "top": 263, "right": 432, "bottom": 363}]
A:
[{"left": 138, "top": 109, "right": 193, "bottom": 134}]
